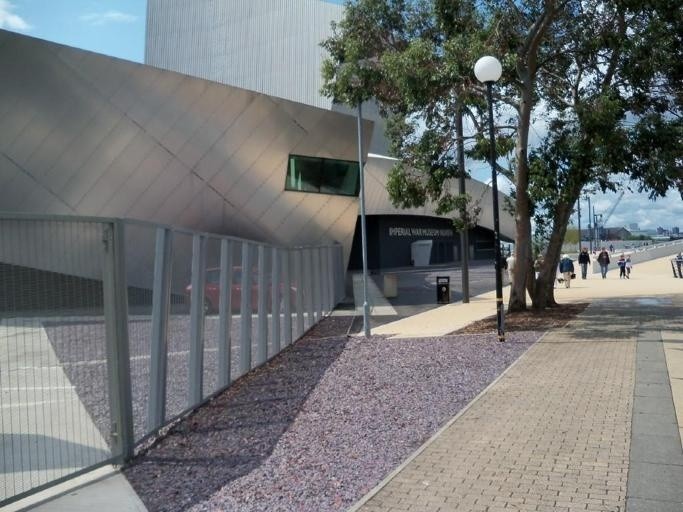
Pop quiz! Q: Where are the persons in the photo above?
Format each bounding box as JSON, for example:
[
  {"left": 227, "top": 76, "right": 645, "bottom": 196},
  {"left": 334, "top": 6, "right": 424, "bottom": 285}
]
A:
[
  {"left": 597, "top": 247, "right": 610, "bottom": 278},
  {"left": 625, "top": 256, "right": 633, "bottom": 279},
  {"left": 578, "top": 247, "right": 590, "bottom": 280},
  {"left": 534, "top": 254, "right": 544, "bottom": 280},
  {"left": 609, "top": 244, "right": 613, "bottom": 252},
  {"left": 617, "top": 254, "right": 626, "bottom": 279},
  {"left": 495, "top": 254, "right": 508, "bottom": 286},
  {"left": 560, "top": 254, "right": 574, "bottom": 288},
  {"left": 506, "top": 252, "right": 516, "bottom": 285}
]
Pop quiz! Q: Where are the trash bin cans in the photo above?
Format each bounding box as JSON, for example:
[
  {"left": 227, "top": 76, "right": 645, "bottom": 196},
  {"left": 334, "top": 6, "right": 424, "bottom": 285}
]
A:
[
  {"left": 383, "top": 275, "right": 398, "bottom": 297},
  {"left": 436, "top": 276, "right": 450, "bottom": 304}
]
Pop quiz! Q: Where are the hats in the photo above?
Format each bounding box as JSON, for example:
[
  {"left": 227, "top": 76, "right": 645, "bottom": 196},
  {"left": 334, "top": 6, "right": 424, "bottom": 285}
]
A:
[{"left": 563, "top": 255, "right": 570, "bottom": 258}]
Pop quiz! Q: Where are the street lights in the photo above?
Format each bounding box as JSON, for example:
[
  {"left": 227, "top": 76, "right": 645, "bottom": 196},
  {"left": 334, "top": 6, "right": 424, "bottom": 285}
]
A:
[
  {"left": 337, "top": 61, "right": 371, "bottom": 336},
  {"left": 472, "top": 57, "right": 505, "bottom": 340},
  {"left": 593, "top": 214, "right": 604, "bottom": 255}
]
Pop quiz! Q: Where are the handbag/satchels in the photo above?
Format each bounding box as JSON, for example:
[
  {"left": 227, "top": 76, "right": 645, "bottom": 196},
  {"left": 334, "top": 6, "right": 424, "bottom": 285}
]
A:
[{"left": 571, "top": 274, "right": 575, "bottom": 279}]
